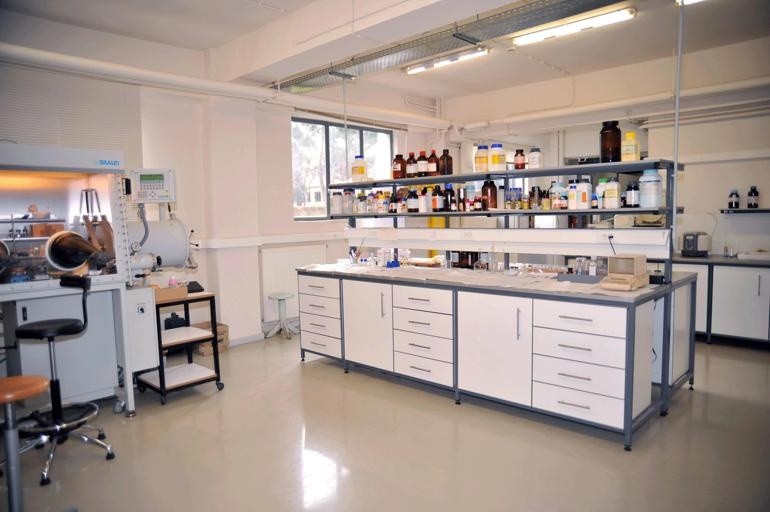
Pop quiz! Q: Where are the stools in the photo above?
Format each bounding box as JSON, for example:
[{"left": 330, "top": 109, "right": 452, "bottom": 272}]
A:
[
  {"left": 0, "top": 375, "right": 49, "bottom": 512},
  {"left": 265, "top": 293, "right": 300, "bottom": 339}
]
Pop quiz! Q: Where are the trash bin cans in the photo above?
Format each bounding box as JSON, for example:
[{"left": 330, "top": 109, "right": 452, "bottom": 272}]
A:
[{"left": 190, "top": 321, "right": 229, "bottom": 356}]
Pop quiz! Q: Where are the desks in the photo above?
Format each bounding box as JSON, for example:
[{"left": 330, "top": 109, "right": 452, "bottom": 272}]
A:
[{"left": 137, "top": 289, "right": 223, "bottom": 407}]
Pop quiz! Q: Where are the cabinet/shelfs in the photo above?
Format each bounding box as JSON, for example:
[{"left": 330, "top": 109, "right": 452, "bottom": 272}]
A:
[
  {"left": 296, "top": 159, "right": 698, "bottom": 453},
  {"left": 563, "top": 255, "right": 770, "bottom": 347},
  {"left": 0, "top": 145, "right": 135, "bottom": 421}
]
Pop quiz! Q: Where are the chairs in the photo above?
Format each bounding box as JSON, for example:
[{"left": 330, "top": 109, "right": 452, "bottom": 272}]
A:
[{"left": 16, "top": 274, "right": 114, "bottom": 488}]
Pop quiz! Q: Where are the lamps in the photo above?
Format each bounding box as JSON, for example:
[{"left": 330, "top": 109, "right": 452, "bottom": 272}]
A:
[
  {"left": 404, "top": 46, "right": 489, "bottom": 77},
  {"left": 510, "top": 6, "right": 639, "bottom": 48}
]
